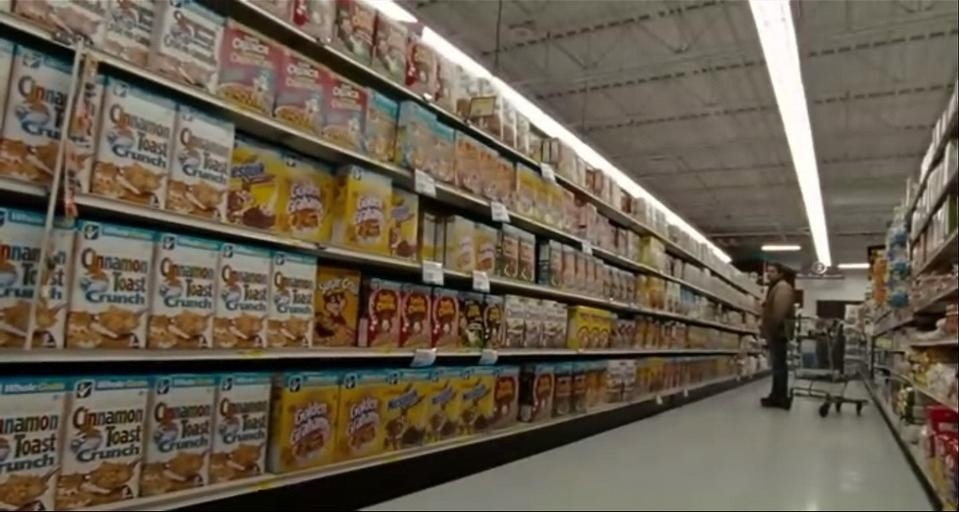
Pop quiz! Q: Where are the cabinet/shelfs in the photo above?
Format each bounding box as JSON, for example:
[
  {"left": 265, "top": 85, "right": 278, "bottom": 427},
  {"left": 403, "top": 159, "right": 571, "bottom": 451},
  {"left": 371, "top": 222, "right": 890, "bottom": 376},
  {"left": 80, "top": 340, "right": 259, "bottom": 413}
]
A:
[
  {"left": 856, "top": 91, "right": 958, "bottom": 512},
  {"left": 2, "top": 1, "right": 773, "bottom": 512}
]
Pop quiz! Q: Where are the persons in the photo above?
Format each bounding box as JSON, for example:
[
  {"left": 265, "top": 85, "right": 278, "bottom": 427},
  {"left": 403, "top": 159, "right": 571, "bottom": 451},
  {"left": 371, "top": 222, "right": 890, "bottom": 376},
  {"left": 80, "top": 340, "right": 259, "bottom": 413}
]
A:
[{"left": 761, "top": 261, "right": 795, "bottom": 409}]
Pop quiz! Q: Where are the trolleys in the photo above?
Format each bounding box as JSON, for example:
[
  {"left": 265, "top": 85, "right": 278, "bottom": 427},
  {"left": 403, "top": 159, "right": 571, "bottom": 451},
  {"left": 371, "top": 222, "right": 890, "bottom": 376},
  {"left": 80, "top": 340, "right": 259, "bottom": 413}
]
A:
[{"left": 788, "top": 312, "right": 872, "bottom": 417}]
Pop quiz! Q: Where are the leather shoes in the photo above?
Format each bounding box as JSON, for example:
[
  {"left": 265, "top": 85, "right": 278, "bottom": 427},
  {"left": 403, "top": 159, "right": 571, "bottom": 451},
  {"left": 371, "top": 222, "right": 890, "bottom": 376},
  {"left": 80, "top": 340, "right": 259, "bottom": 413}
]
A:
[
  {"left": 773, "top": 398, "right": 789, "bottom": 409},
  {"left": 761, "top": 393, "right": 774, "bottom": 406}
]
